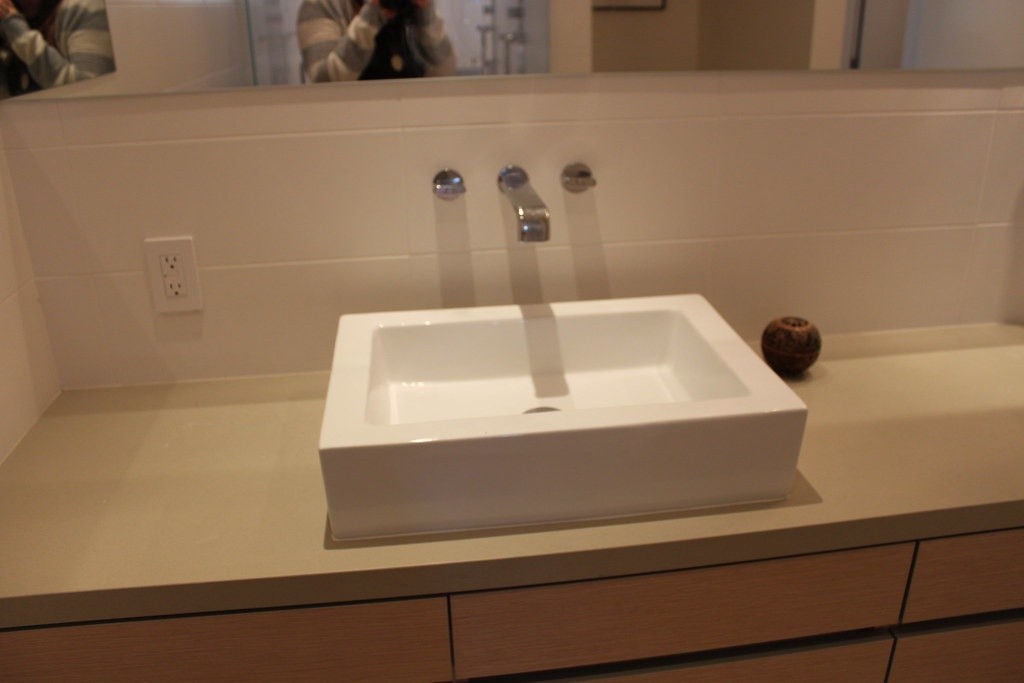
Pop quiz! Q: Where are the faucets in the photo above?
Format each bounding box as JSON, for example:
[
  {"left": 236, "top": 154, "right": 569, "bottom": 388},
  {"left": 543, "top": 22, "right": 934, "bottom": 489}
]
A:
[{"left": 497, "top": 163, "right": 551, "bottom": 242}]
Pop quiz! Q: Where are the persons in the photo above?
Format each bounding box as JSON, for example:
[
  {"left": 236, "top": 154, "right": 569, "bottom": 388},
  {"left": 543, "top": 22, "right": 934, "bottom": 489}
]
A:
[
  {"left": 296, "top": 0, "right": 458, "bottom": 84},
  {"left": 0, "top": 0, "right": 117, "bottom": 102}
]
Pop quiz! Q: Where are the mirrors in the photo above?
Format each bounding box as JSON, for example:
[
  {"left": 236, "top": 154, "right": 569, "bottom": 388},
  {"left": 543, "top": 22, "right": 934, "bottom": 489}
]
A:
[
  {"left": 1, "top": 0, "right": 1022, "bottom": 102},
  {"left": 0, "top": 0, "right": 117, "bottom": 102}
]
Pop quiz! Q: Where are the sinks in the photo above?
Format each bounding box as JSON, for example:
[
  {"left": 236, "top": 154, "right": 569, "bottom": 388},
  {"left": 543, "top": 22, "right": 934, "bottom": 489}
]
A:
[{"left": 318, "top": 292, "right": 810, "bottom": 542}]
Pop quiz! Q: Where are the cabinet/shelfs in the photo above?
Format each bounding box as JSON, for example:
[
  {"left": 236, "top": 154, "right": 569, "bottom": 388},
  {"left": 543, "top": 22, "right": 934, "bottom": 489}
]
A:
[{"left": 0, "top": 522, "right": 1022, "bottom": 683}]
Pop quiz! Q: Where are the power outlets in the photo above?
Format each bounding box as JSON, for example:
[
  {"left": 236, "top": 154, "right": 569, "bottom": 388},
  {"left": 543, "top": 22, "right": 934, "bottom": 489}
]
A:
[{"left": 144, "top": 234, "right": 205, "bottom": 316}]
